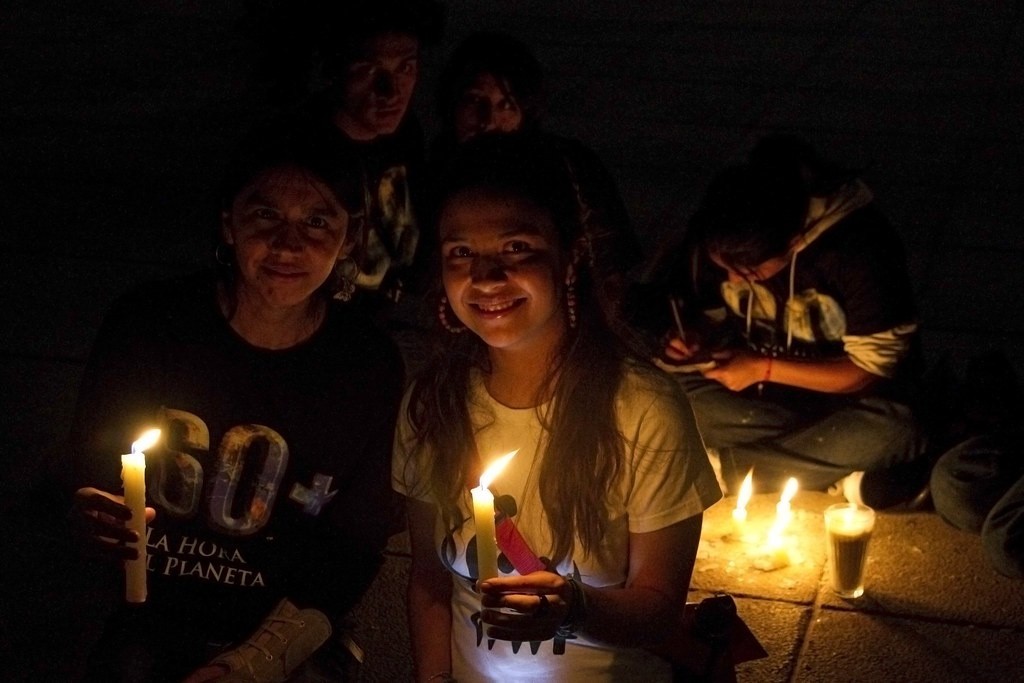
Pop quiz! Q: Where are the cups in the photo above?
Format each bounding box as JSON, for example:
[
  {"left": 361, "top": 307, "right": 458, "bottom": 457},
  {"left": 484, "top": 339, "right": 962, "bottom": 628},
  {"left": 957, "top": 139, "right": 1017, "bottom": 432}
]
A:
[{"left": 823, "top": 499, "right": 875, "bottom": 599}]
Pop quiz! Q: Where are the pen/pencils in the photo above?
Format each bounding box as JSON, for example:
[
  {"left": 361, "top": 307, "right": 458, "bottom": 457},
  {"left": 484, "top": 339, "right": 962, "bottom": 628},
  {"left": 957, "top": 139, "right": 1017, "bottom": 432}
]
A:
[{"left": 671, "top": 299, "right": 689, "bottom": 346}]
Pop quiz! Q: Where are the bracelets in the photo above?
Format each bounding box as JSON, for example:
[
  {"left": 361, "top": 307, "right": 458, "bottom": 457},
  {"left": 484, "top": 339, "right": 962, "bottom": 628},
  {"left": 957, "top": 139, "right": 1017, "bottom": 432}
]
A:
[
  {"left": 559, "top": 576, "right": 586, "bottom": 639},
  {"left": 758, "top": 355, "right": 772, "bottom": 383},
  {"left": 427, "top": 671, "right": 450, "bottom": 682}
]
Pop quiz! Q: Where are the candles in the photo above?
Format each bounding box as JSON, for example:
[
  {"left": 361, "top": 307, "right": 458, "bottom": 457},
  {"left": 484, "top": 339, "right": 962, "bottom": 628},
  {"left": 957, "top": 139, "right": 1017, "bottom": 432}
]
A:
[
  {"left": 734, "top": 465, "right": 800, "bottom": 570},
  {"left": 471, "top": 448, "right": 518, "bottom": 640},
  {"left": 121, "top": 428, "right": 161, "bottom": 603},
  {"left": 825, "top": 502, "right": 876, "bottom": 599}
]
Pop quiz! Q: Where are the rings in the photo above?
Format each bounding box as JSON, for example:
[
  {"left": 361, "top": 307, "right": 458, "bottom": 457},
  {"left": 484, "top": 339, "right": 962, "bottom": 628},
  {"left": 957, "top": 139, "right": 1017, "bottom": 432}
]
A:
[{"left": 535, "top": 592, "right": 548, "bottom": 615}]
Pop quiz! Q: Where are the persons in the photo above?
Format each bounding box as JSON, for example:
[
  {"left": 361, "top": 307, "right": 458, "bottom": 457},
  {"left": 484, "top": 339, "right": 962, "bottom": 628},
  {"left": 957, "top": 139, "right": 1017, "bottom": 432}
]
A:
[
  {"left": 66, "top": 113, "right": 401, "bottom": 682},
  {"left": 648, "top": 136, "right": 929, "bottom": 510},
  {"left": 428, "top": 35, "right": 641, "bottom": 273},
  {"left": 262, "top": 3, "right": 444, "bottom": 307},
  {"left": 388, "top": 132, "right": 724, "bottom": 682},
  {"left": 931, "top": 423, "right": 1024, "bottom": 580}
]
